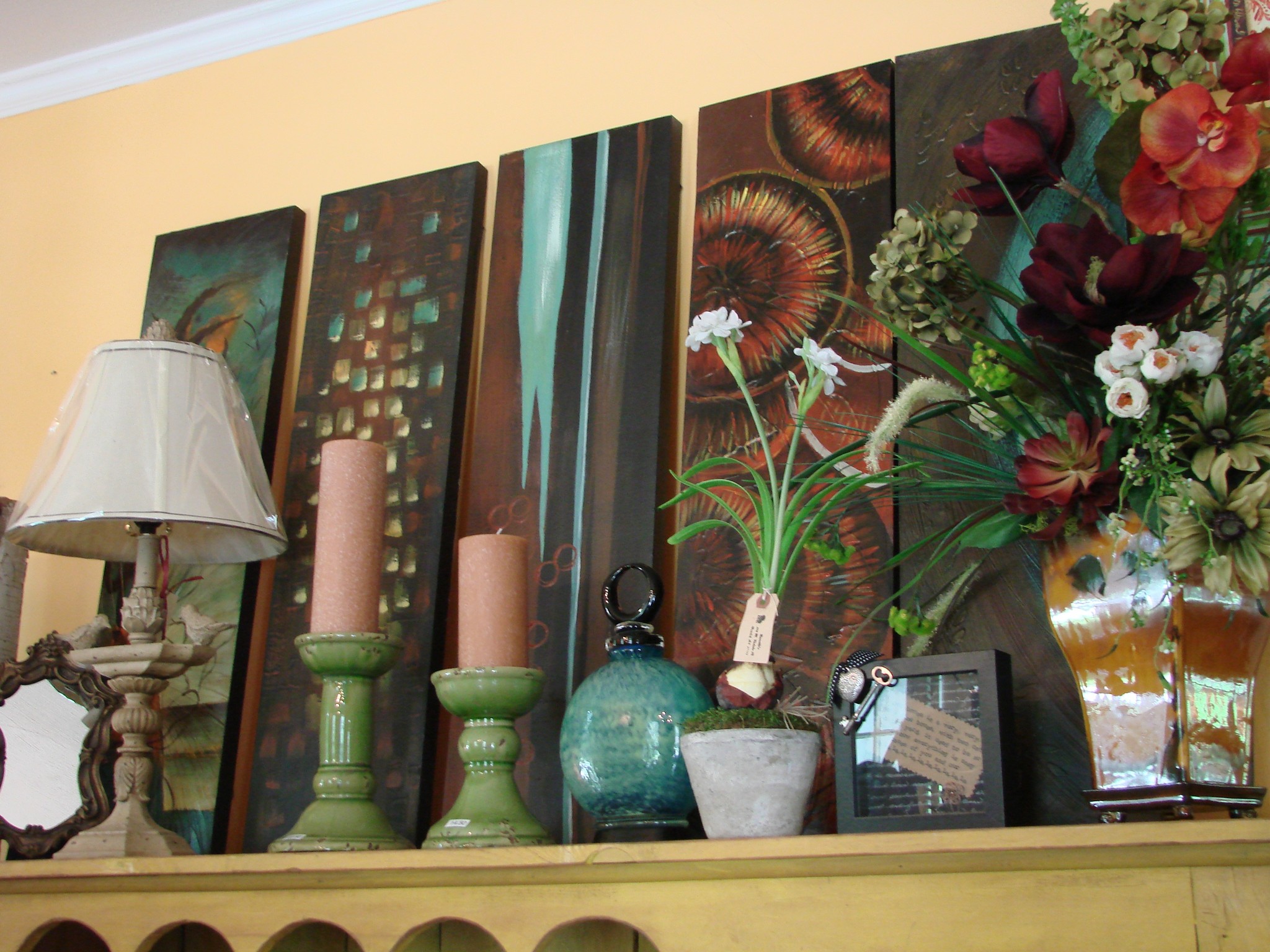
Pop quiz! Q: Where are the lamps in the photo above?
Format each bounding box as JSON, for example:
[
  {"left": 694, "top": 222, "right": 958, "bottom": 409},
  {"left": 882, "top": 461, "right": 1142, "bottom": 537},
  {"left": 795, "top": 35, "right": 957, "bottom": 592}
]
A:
[{"left": 8, "top": 339, "right": 291, "bottom": 865}]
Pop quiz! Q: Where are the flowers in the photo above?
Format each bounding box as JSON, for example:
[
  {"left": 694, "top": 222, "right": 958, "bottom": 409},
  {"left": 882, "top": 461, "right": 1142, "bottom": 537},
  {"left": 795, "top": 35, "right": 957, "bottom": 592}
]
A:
[
  {"left": 802, "top": 0, "right": 1270, "bottom": 662},
  {"left": 652, "top": 305, "right": 940, "bottom": 709}
]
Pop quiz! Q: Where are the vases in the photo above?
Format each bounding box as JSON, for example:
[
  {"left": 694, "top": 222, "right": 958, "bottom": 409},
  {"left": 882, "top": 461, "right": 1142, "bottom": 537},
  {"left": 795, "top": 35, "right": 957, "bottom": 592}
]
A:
[
  {"left": 1039, "top": 504, "right": 1269, "bottom": 829},
  {"left": 680, "top": 704, "right": 831, "bottom": 843}
]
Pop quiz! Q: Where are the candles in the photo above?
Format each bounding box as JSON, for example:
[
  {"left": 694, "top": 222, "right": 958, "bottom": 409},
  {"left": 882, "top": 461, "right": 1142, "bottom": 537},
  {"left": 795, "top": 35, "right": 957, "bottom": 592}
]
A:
[
  {"left": 453, "top": 527, "right": 536, "bottom": 672},
  {"left": 306, "top": 437, "right": 391, "bottom": 635}
]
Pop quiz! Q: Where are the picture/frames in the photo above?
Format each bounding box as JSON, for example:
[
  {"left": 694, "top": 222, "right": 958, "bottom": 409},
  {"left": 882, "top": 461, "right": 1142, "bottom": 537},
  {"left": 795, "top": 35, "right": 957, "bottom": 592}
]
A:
[{"left": 827, "top": 643, "right": 1015, "bottom": 834}]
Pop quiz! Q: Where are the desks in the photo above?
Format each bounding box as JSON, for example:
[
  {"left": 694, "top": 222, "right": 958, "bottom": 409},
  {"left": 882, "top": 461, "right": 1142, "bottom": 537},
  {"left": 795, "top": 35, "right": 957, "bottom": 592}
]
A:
[{"left": 0, "top": 812, "right": 1270, "bottom": 952}]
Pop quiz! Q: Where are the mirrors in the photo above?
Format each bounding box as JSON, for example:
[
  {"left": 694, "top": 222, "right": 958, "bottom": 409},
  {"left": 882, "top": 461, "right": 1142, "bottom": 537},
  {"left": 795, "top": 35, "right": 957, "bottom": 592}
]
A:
[{"left": 0, "top": 626, "right": 127, "bottom": 866}]
